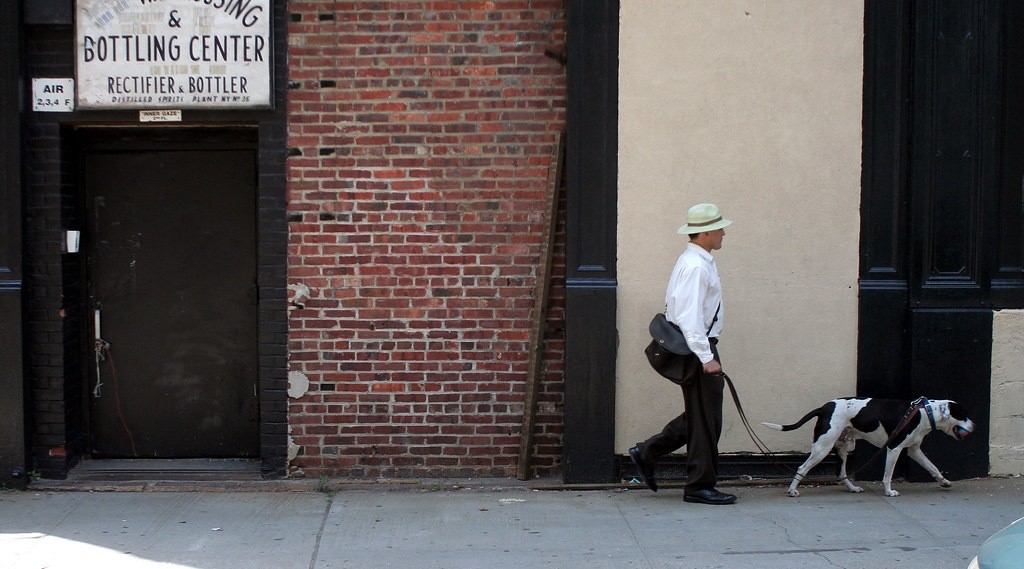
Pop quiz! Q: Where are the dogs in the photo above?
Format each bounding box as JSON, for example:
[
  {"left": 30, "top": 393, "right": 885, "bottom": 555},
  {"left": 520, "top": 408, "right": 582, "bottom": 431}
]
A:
[{"left": 760, "top": 396, "right": 975, "bottom": 497}]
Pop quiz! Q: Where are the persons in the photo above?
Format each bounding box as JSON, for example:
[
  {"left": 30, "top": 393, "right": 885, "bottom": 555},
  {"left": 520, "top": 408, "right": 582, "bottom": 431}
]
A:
[{"left": 629, "top": 203, "right": 737, "bottom": 504}]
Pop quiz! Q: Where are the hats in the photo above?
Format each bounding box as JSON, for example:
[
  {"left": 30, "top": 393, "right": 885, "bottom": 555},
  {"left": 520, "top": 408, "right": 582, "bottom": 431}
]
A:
[{"left": 677, "top": 204, "right": 733, "bottom": 234}]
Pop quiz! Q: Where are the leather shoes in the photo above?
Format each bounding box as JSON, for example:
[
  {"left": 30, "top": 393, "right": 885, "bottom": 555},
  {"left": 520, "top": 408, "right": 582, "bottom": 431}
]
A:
[
  {"left": 684, "top": 486, "right": 737, "bottom": 504},
  {"left": 629, "top": 446, "right": 658, "bottom": 492}
]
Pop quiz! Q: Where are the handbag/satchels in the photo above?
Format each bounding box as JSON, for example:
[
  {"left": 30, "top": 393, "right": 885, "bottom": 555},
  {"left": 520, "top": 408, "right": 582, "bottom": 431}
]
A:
[{"left": 644, "top": 313, "right": 701, "bottom": 386}]
